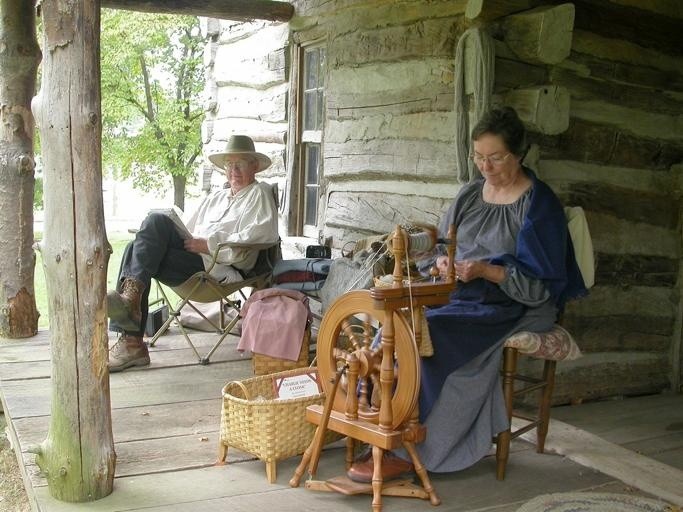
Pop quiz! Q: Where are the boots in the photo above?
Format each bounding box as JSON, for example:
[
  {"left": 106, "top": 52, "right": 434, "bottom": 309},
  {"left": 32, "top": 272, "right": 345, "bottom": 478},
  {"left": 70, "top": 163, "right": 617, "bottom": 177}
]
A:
[
  {"left": 108, "top": 333, "right": 150, "bottom": 371},
  {"left": 108, "top": 276, "right": 146, "bottom": 332}
]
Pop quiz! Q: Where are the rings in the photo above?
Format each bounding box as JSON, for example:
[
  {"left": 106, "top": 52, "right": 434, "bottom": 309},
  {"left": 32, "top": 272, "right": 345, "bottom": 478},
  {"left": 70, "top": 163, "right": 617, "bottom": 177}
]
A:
[{"left": 456, "top": 275, "right": 460, "bottom": 281}]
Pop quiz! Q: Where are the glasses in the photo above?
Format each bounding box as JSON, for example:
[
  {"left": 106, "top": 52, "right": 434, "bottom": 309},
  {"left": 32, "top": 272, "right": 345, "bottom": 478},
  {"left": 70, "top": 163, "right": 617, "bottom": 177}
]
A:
[
  {"left": 469, "top": 150, "right": 512, "bottom": 164},
  {"left": 224, "top": 159, "right": 251, "bottom": 171}
]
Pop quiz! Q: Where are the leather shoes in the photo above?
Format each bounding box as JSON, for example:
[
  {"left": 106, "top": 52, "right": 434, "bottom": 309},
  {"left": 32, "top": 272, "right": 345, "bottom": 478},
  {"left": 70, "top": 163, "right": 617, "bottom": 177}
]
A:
[{"left": 346, "top": 451, "right": 418, "bottom": 484}]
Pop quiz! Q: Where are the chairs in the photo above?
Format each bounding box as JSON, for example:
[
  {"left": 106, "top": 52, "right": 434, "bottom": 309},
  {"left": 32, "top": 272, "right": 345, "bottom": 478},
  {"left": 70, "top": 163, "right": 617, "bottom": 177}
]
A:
[
  {"left": 491, "top": 206, "right": 595, "bottom": 480},
  {"left": 146, "top": 182, "right": 279, "bottom": 365}
]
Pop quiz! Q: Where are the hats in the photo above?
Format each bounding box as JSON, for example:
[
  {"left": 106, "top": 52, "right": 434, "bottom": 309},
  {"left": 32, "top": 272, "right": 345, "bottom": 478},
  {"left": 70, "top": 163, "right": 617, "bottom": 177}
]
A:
[{"left": 208, "top": 135, "right": 271, "bottom": 172}]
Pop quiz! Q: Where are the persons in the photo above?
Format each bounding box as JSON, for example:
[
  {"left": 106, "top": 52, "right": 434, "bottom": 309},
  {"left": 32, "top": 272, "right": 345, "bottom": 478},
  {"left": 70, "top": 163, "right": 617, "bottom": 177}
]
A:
[
  {"left": 344, "top": 104, "right": 589, "bottom": 486},
  {"left": 107, "top": 135, "right": 280, "bottom": 376}
]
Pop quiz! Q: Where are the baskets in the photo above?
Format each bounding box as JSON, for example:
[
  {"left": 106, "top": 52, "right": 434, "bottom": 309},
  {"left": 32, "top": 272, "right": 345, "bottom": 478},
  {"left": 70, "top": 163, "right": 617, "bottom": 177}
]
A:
[
  {"left": 253, "top": 328, "right": 311, "bottom": 375},
  {"left": 220, "top": 366, "right": 347, "bottom": 463}
]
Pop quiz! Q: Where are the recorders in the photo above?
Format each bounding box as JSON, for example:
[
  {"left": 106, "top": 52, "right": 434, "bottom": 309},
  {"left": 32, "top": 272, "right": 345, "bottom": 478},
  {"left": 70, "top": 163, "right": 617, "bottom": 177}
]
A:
[{"left": 146, "top": 298, "right": 168, "bottom": 337}]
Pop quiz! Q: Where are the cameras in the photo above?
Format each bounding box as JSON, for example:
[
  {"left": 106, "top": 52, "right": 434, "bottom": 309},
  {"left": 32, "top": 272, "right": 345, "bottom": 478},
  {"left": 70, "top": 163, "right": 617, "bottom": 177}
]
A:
[{"left": 306, "top": 246, "right": 330, "bottom": 258}]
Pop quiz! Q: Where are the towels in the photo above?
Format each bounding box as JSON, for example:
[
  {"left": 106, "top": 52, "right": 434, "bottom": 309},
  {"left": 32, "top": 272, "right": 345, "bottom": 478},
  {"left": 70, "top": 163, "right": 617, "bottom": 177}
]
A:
[
  {"left": 286, "top": 282, "right": 325, "bottom": 292},
  {"left": 272, "top": 256, "right": 333, "bottom": 275},
  {"left": 276, "top": 270, "right": 328, "bottom": 284}
]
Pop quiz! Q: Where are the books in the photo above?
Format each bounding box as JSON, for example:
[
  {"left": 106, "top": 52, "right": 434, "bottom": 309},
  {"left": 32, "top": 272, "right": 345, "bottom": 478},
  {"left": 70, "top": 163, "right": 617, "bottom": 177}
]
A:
[
  {"left": 150, "top": 207, "right": 193, "bottom": 240},
  {"left": 272, "top": 371, "right": 322, "bottom": 398}
]
